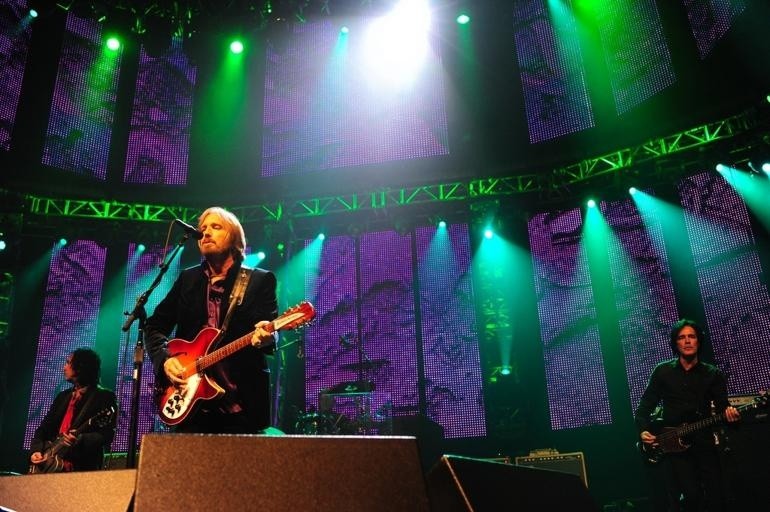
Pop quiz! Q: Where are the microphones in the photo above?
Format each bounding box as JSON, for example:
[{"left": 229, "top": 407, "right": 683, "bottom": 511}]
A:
[{"left": 175, "top": 218, "right": 203, "bottom": 240}]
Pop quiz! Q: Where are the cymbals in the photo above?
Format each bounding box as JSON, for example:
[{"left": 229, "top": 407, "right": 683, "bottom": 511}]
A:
[
  {"left": 323, "top": 381, "right": 374, "bottom": 395},
  {"left": 343, "top": 358, "right": 385, "bottom": 373}
]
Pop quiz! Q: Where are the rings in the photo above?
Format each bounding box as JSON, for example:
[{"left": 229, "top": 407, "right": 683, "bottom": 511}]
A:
[{"left": 257, "top": 341, "right": 262, "bottom": 348}]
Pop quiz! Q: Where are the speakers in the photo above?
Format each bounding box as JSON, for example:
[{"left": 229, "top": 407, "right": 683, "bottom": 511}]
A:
[
  {"left": 134, "top": 433, "right": 430, "bottom": 511},
  {"left": 0, "top": 469, "right": 138, "bottom": 512},
  {"left": 427, "top": 454, "right": 595, "bottom": 512},
  {"left": 515, "top": 451, "right": 588, "bottom": 489}
]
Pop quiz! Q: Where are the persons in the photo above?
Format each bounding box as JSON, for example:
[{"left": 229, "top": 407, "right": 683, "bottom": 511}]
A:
[
  {"left": 30, "top": 349, "right": 119, "bottom": 472},
  {"left": 143, "top": 207, "right": 278, "bottom": 434},
  {"left": 635, "top": 319, "right": 742, "bottom": 512}
]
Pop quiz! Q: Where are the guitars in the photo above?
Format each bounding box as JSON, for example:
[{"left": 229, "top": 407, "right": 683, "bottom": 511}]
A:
[
  {"left": 152, "top": 301, "right": 317, "bottom": 428},
  {"left": 636, "top": 390, "right": 770, "bottom": 463},
  {"left": 27, "top": 405, "right": 115, "bottom": 474}
]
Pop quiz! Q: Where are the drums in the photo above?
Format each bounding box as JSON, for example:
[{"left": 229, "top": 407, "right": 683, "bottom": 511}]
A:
[
  {"left": 295, "top": 412, "right": 347, "bottom": 436},
  {"left": 377, "top": 414, "right": 443, "bottom": 468}
]
